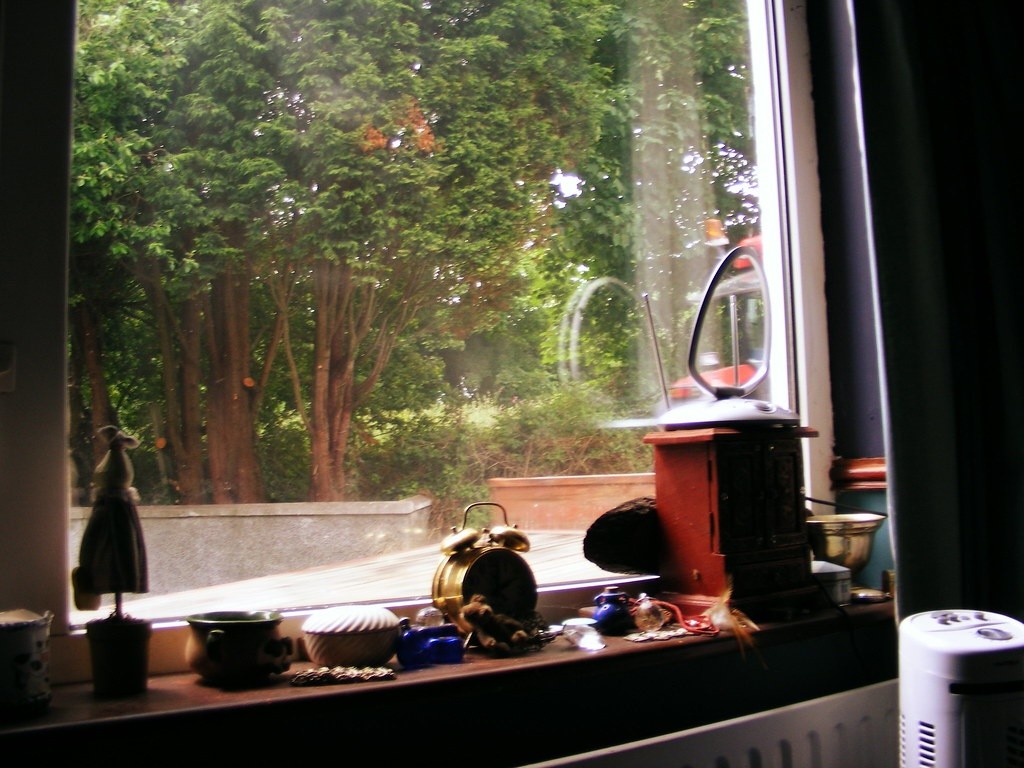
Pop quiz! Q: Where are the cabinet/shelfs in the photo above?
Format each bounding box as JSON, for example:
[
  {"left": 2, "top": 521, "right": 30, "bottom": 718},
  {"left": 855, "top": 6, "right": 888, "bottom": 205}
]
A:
[{"left": 644, "top": 428, "right": 821, "bottom": 626}]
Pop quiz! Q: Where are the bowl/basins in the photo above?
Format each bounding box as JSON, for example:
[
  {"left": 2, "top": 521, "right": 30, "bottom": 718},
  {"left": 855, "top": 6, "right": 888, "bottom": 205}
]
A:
[
  {"left": 301, "top": 626, "right": 404, "bottom": 669},
  {"left": 804, "top": 512, "right": 887, "bottom": 573}
]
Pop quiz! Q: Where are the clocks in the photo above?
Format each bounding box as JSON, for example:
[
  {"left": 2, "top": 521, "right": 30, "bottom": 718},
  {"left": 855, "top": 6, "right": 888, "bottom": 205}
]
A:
[{"left": 432, "top": 501, "right": 539, "bottom": 644}]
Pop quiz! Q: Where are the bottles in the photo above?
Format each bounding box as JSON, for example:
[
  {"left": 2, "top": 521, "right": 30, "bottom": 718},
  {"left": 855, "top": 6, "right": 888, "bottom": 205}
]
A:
[{"left": 184, "top": 611, "right": 286, "bottom": 684}]
